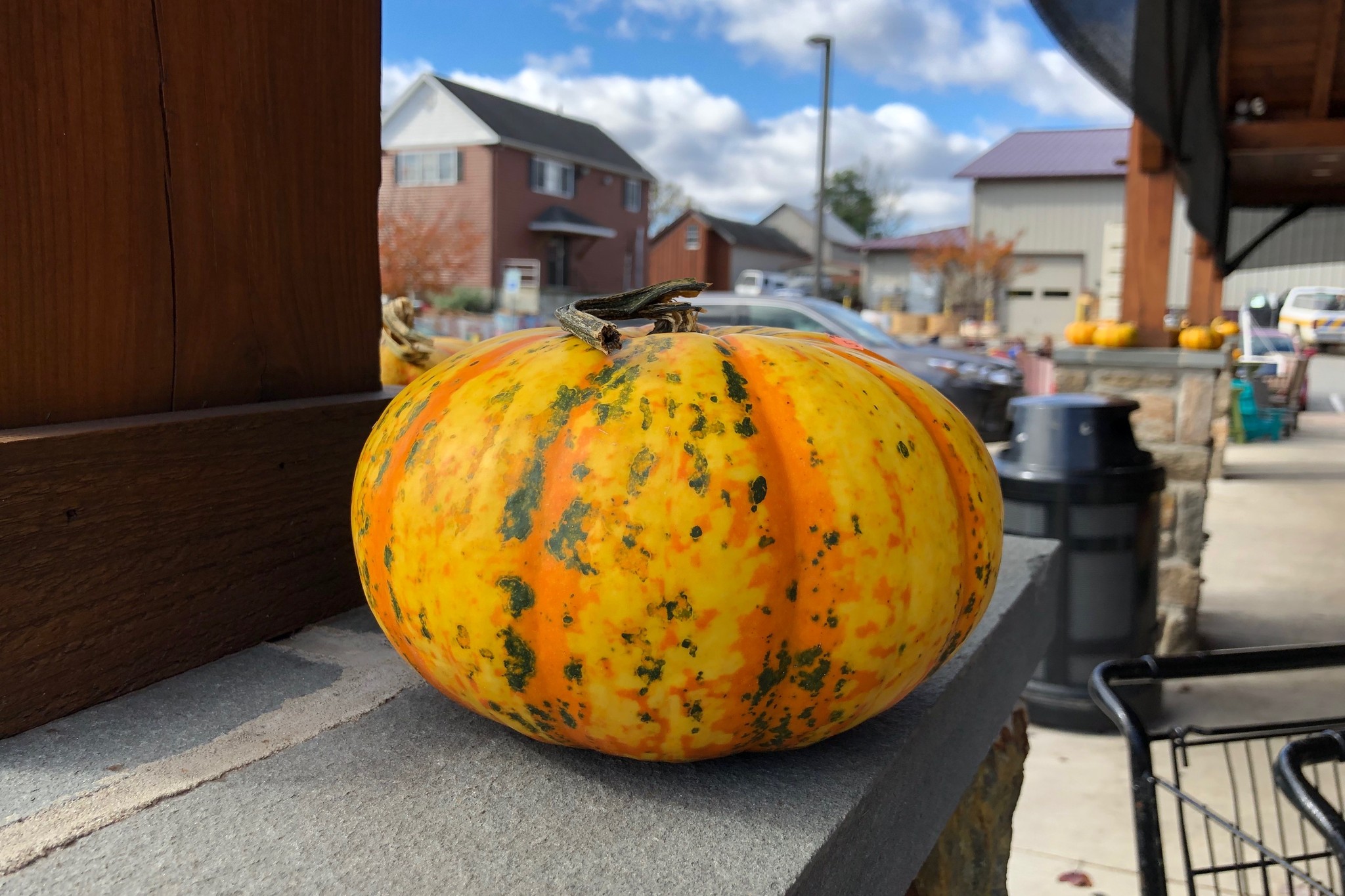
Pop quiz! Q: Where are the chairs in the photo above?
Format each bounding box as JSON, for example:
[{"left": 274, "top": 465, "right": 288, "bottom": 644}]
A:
[
  {"left": 1253, "top": 359, "right": 1309, "bottom": 433},
  {"left": 1232, "top": 378, "right": 1293, "bottom": 441}
]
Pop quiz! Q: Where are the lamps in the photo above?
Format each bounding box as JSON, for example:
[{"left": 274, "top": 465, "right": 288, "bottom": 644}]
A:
[{"left": 1234, "top": 96, "right": 1270, "bottom": 117}]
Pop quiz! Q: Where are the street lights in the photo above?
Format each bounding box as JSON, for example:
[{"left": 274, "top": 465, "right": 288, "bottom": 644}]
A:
[{"left": 808, "top": 36, "right": 832, "bottom": 297}]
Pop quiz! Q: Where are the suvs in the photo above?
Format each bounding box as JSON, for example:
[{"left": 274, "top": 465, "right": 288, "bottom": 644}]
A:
[{"left": 666, "top": 290, "right": 1025, "bottom": 443}]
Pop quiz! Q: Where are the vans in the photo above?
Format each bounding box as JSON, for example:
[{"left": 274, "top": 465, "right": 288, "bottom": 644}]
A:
[{"left": 733, "top": 269, "right": 792, "bottom": 297}]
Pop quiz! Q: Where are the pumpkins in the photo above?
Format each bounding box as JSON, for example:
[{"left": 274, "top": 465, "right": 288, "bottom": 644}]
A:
[
  {"left": 349, "top": 278, "right": 1006, "bottom": 764},
  {"left": 1232, "top": 347, "right": 1243, "bottom": 358},
  {"left": 1178, "top": 317, "right": 1239, "bottom": 349},
  {"left": 1063, "top": 304, "right": 1142, "bottom": 348}
]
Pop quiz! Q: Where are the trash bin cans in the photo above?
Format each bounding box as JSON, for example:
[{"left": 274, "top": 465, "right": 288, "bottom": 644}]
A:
[{"left": 987, "top": 393, "right": 1167, "bottom": 735}]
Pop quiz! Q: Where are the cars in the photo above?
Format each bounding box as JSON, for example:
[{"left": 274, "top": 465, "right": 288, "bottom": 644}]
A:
[
  {"left": 1277, "top": 286, "right": 1345, "bottom": 354},
  {"left": 1221, "top": 325, "right": 1321, "bottom": 412}
]
